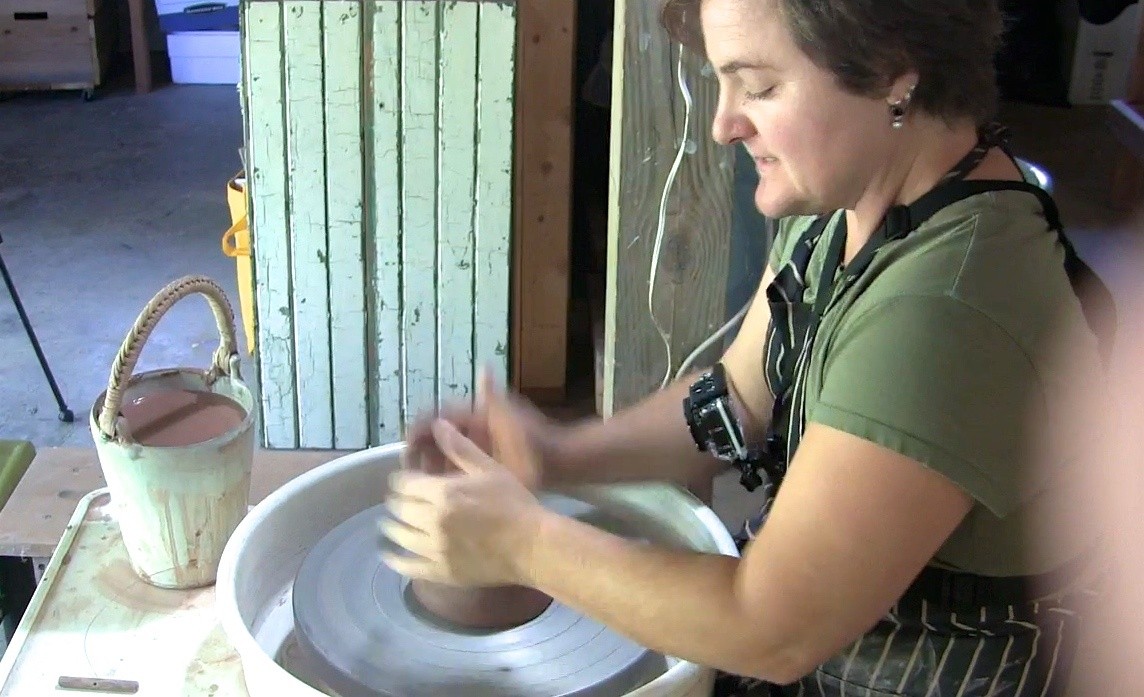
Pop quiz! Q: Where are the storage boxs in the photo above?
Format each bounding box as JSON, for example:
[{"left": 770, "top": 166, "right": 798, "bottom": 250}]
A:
[
  {"left": 165, "top": 31, "right": 242, "bottom": 85},
  {"left": 1061, "top": 0, "right": 1144, "bottom": 105}
]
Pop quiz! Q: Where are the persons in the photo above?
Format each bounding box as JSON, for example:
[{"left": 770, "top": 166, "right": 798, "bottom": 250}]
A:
[{"left": 375, "top": 1, "right": 1143, "bottom": 695}]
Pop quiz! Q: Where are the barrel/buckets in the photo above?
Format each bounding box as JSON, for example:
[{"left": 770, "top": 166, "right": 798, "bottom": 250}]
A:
[{"left": 89, "top": 275, "right": 264, "bottom": 590}]
